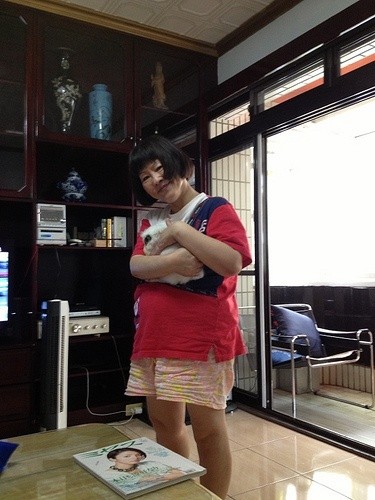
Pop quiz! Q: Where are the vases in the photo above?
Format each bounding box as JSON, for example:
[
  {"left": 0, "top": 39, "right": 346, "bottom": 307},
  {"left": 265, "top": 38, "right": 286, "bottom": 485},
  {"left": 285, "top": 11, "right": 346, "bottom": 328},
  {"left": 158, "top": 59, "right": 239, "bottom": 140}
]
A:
[
  {"left": 48, "top": 47, "right": 84, "bottom": 134},
  {"left": 87, "top": 83, "right": 112, "bottom": 141}
]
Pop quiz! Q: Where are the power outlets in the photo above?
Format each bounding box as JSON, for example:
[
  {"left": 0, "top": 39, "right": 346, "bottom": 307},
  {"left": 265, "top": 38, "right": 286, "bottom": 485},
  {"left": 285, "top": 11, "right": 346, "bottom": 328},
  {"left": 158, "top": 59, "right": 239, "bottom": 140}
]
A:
[{"left": 126, "top": 402, "right": 143, "bottom": 416}]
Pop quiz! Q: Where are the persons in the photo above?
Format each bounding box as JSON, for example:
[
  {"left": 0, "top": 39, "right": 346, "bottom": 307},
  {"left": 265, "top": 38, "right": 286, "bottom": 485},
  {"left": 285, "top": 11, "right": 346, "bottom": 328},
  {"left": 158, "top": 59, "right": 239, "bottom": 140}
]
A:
[
  {"left": 124, "top": 137, "right": 253, "bottom": 500},
  {"left": 150, "top": 62, "right": 167, "bottom": 106},
  {"left": 103, "top": 448, "right": 196, "bottom": 492}
]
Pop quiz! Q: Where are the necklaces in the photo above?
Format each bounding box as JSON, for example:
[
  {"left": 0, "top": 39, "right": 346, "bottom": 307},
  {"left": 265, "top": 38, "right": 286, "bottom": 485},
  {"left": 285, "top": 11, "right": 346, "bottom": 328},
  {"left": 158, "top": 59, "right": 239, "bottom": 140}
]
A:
[{"left": 111, "top": 465, "right": 138, "bottom": 472}]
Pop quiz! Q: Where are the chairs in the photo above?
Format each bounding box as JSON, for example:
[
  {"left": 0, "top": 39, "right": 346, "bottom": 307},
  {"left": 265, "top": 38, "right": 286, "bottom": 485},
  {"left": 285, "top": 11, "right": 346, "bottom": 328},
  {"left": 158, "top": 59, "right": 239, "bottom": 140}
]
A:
[{"left": 239, "top": 304, "right": 374, "bottom": 420}]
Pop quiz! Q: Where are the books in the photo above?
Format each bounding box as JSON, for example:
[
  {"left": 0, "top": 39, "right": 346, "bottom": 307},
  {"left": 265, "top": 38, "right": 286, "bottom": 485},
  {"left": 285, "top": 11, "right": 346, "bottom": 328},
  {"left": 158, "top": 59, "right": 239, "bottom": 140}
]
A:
[
  {"left": 72, "top": 436, "right": 207, "bottom": 500},
  {"left": 100, "top": 216, "right": 126, "bottom": 247}
]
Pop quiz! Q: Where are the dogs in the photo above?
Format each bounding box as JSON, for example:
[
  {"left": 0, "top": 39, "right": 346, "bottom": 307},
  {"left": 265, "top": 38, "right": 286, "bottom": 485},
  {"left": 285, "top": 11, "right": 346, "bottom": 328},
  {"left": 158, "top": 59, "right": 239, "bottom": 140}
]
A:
[{"left": 138, "top": 221, "right": 206, "bottom": 285}]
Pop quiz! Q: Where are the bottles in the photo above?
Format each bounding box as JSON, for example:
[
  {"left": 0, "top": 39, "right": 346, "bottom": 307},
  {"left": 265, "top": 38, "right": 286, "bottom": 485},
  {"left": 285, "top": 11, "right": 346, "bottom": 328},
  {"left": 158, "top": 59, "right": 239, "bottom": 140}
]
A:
[
  {"left": 48, "top": 46, "right": 81, "bottom": 133},
  {"left": 56, "top": 168, "right": 88, "bottom": 202},
  {"left": 89, "top": 84, "right": 112, "bottom": 141}
]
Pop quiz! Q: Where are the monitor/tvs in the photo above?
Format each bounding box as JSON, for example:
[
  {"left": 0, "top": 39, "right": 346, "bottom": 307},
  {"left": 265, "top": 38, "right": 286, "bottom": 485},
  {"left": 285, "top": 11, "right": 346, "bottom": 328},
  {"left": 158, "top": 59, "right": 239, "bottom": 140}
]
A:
[{"left": 0, "top": 240, "right": 16, "bottom": 337}]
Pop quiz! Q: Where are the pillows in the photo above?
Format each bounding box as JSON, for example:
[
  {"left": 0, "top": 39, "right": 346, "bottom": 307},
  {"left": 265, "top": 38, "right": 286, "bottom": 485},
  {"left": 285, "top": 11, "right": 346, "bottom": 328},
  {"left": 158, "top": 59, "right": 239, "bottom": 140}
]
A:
[{"left": 271, "top": 305, "right": 324, "bottom": 357}]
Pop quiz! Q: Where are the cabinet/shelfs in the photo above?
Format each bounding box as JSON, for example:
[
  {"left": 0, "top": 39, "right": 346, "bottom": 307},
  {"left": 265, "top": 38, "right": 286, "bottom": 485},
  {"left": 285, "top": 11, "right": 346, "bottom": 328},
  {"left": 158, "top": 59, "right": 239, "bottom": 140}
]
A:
[{"left": 0, "top": 0, "right": 210, "bottom": 434}]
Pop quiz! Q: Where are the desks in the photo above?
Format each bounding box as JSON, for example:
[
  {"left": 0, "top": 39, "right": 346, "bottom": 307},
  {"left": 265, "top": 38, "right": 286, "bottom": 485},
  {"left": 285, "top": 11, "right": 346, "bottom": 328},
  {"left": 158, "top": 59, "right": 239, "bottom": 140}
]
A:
[{"left": 0, "top": 424, "right": 223, "bottom": 500}]
